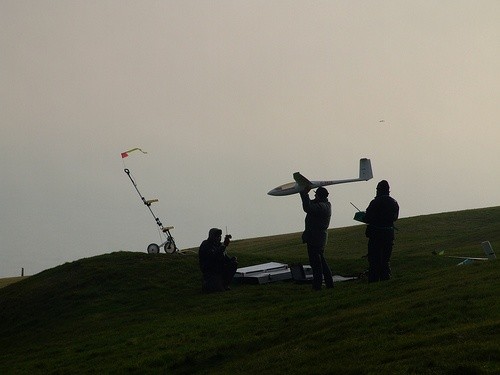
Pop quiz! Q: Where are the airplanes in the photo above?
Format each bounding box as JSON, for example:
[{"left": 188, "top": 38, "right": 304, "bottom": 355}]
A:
[{"left": 267, "top": 157, "right": 374, "bottom": 196}]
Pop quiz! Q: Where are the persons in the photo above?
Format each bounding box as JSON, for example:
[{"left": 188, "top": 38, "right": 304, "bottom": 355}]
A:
[
  {"left": 362, "top": 180, "right": 399, "bottom": 283},
  {"left": 198, "top": 228, "right": 238, "bottom": 292},
  {"left": 300, "top": 186, "right": 334, "bottom": 290}
]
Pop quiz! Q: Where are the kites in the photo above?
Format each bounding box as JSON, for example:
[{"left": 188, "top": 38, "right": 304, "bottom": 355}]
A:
[{"left": 121, "top": 147, "right": 148, "bottom": 158}]
